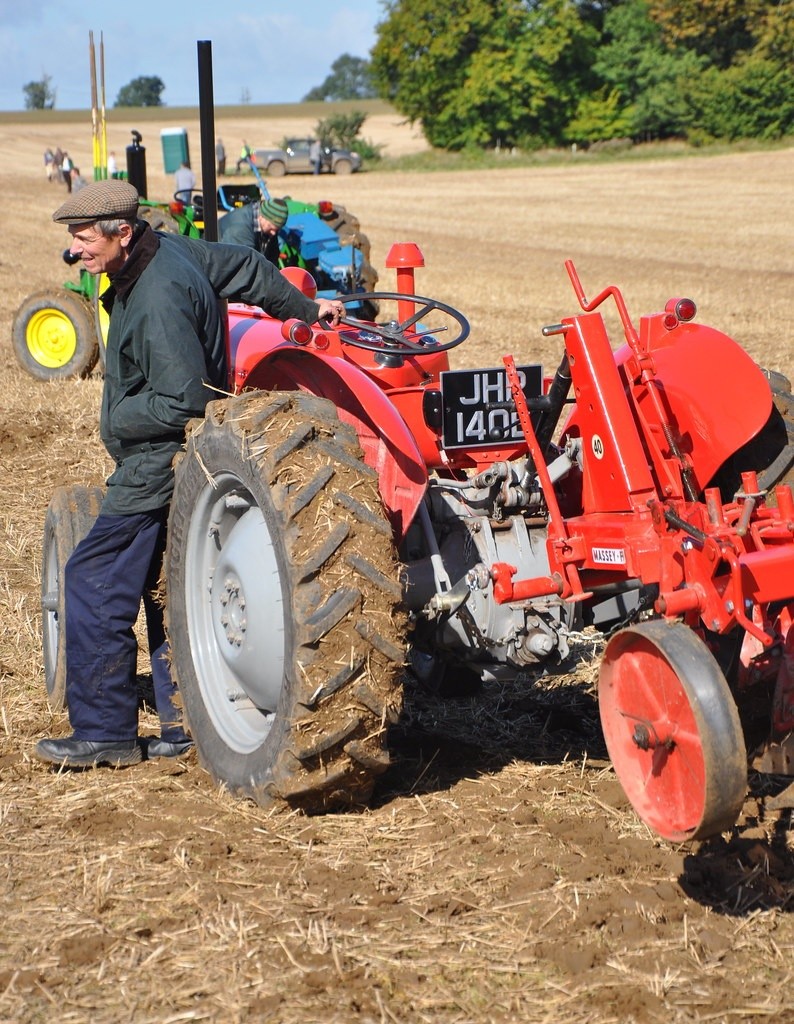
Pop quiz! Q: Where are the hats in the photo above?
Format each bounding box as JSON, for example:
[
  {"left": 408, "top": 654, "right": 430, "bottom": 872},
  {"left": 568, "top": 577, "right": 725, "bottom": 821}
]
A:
[
  {"left": 258, "top": 198, "right": 288, "bottom": 228},
  {"left": 52, "top": 179, "right": 139, "bottom": 224}
]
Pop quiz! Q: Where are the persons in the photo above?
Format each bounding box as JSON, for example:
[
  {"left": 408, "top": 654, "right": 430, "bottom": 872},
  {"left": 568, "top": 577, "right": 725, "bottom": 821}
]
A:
[
  {"left": 237, "top": 139, "right": 254, "bottom": 171},
  {"left": 174, "top": 161, "right": 195, "bottom": 203},
  {"left": 216, "top": 139, "right": 226, "bottom": 176},
  {"left": 36, "top": 179, "right": 349, "bottom": 767},
  {"left": 217, "top": 199, "right": 289, "bottom": 270},
  {"left": 106, "top": 151, "right": 117, "bottom": 174},
  {"left": 310, "top": 140, "right": 322, "bottom": 174},
  {"left": 44, "top": 146, "right": 80, "bottom": 192}
]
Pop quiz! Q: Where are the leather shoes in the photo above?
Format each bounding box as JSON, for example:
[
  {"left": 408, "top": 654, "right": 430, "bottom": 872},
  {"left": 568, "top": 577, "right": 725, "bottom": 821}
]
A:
[
  {"left": 36, "top": 734, "right": 141, "bottom": 767},
  {"left": 137, "top": 735, "right": 195, "bottom": 760}
]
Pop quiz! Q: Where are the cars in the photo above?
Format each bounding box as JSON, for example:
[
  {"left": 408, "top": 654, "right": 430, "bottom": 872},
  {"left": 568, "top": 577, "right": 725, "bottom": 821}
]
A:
[{"left": 251, "top": 138, "right": 363, "bottom": 176}]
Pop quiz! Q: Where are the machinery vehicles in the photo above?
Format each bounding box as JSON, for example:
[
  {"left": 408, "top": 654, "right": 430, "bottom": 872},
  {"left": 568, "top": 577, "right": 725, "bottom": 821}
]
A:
[
  {"left": 9, "top": 125, "right": 431, "bottom": 400},
  {"left": 34, "top": 40, "right": 791, "bottom": 850}
]
